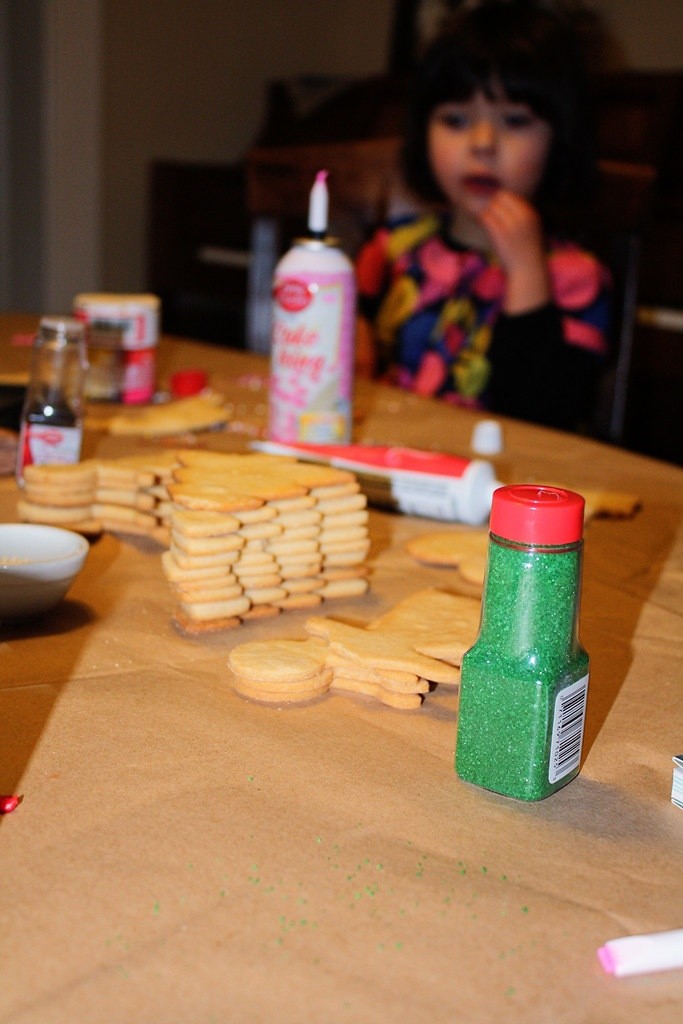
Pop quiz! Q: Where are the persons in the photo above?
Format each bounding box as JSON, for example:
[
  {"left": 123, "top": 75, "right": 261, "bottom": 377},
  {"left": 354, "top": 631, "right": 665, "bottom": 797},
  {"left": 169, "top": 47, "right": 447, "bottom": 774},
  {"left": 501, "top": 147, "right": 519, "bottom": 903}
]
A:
[{"left": 352, "top": 0, "right": 627, "bottom": 448}]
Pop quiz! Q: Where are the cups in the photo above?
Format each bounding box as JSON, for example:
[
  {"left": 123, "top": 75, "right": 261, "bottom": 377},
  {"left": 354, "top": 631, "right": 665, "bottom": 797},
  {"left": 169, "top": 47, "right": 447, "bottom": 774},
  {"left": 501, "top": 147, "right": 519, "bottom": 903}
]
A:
[{"left": 73, "top": 294, "right": 162, "bottom": 405}]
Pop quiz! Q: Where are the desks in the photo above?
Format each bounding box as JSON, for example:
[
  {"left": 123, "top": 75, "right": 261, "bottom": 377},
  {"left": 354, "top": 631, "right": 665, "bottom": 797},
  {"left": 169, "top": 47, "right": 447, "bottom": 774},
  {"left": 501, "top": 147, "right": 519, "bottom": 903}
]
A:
[{"left": 0, "top": 316, "right": 683, "bottom": 1024}]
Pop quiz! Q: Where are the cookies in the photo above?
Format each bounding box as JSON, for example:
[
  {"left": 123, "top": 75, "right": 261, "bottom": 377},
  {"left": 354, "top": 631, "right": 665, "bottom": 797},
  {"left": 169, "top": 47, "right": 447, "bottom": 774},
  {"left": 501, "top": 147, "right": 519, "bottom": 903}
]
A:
[
  {"left": 537, "top": 482, "right": 639, "bottom": 524},
  {"left": 15, "top": 448, "right": 488, "bottom": 710}
]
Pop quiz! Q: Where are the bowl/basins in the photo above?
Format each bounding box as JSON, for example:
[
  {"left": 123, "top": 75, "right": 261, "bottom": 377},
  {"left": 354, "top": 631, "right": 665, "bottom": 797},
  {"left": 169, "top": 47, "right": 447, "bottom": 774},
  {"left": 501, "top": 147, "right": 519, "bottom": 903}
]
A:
[{"left": 0, "top": 522, "right": 91, "bottom": 616}]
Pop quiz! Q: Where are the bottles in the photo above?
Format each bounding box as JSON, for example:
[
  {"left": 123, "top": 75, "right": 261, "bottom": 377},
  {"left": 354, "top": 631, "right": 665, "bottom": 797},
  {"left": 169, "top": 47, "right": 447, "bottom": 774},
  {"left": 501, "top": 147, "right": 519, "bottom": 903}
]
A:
[
  {"left": 15, "top": 314, "right": 92, "bottom": 490},
  {"left": 453, "top": 484, "right": 592, "bottom": 805},
  {"left": 268, "top": 238, "right": 358, "bottom": 450}
]
